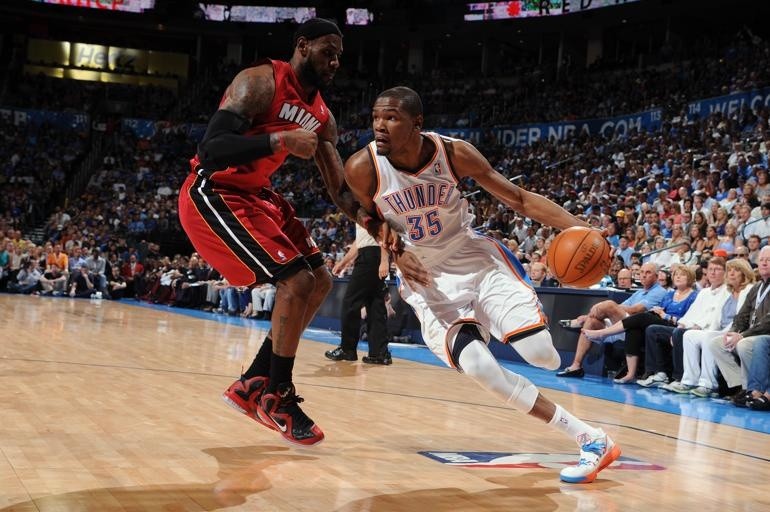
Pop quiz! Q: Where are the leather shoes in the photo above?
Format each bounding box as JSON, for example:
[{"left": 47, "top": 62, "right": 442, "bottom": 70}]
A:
[
  {"left": 556, "top": 319, "right": 604, "bottom": 378},
  {"left": 325, "top": 347, "right": 391, "bottom": 365}
]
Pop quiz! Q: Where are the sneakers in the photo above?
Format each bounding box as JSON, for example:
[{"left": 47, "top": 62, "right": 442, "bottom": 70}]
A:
[
  {"left": 613, "top": 373, "right": 770, "bottom": 411},
  {"left": 220, "top": 374, "right": 325, "bottom": 449},
  {"left": 560, "top": 427, "right": 622, "bottom": 483}
]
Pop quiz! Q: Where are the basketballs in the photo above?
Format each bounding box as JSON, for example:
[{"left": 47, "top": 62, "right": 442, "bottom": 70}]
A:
[{"left": 549, "top": 226, "right": 611, "bottom": 287}]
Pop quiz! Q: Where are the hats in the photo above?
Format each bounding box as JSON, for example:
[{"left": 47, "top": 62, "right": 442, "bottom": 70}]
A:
[
  {"left": 614, "top": 210, "right": 626, "bottom": 218},
  {"left": 713, "top": 249, "right": 728, "bottom": 258}
]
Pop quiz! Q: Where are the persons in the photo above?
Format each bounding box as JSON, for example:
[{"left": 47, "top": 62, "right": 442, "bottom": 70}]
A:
[
  {"left": 179, "top": 16, "right": 432, "bottom": 447},
  {"left": 343, "top": 85, "right": 621, "bottom": 485},
  {"left": 2, "top": 24, "right": 770, "bottom": 412}
]
